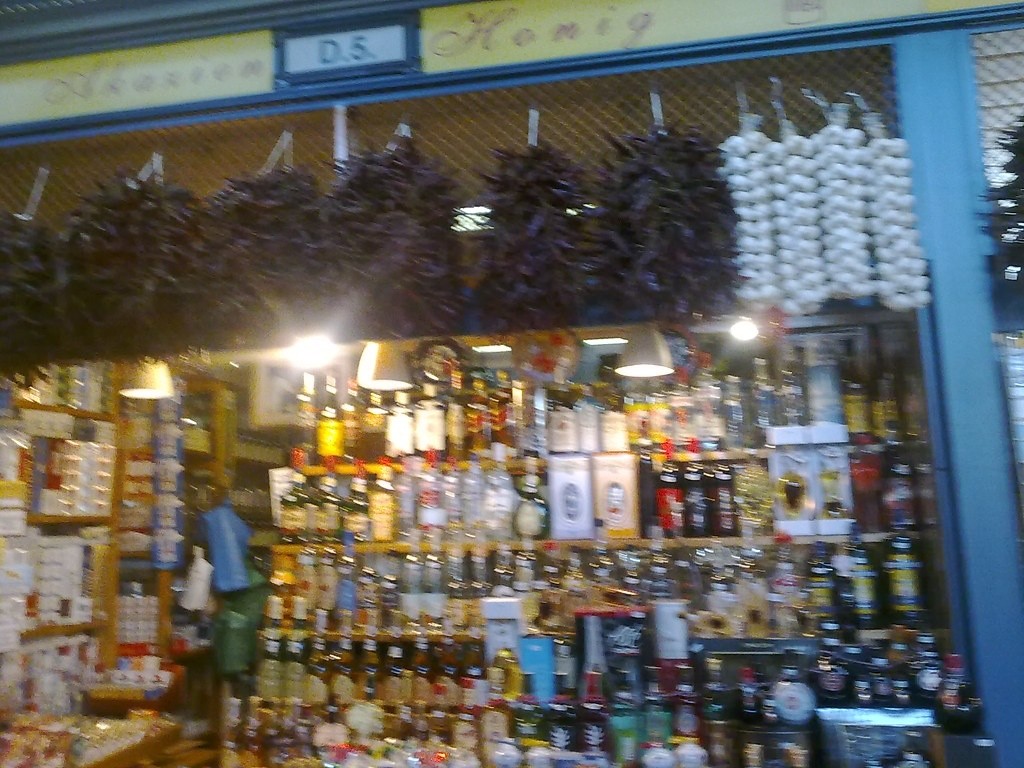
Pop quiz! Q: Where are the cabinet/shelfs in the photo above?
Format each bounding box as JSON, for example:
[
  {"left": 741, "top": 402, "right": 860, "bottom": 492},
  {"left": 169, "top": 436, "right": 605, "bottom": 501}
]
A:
[
  {"left": 13, "top": 397, "right": 117, "bottom": 641},
  {"left": 258, "top": 443, "right": 942, "bottom": 646}
]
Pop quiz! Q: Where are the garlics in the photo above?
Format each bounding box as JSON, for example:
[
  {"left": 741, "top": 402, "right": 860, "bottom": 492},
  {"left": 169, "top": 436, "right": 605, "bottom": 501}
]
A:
[{"left": 715, "top": 123, "right": 931, "bottom": 315}]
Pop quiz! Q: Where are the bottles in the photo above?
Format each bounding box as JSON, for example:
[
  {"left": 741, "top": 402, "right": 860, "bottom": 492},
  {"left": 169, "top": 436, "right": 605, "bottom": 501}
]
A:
[{"left": 215, "top": 358, "right": 984, "bottom": 768}]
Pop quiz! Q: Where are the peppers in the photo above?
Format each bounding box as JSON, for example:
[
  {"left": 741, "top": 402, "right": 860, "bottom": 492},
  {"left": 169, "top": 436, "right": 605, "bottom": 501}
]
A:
[{"left": 0, "top": 120, "right": 750, "bottom": 388}]
